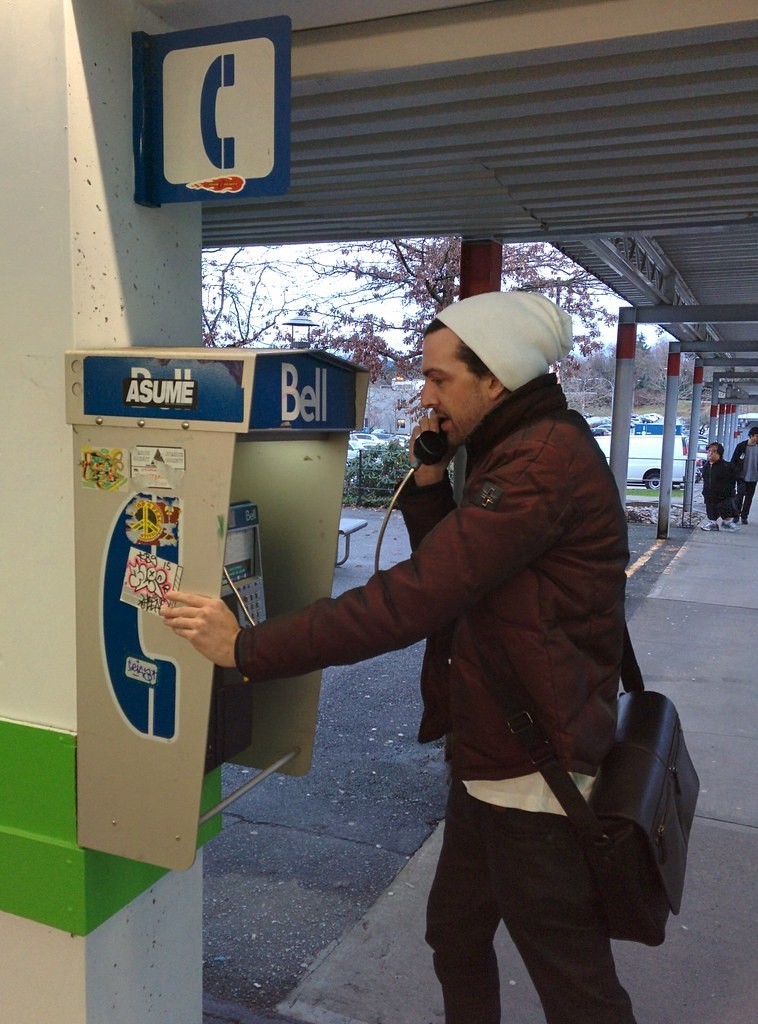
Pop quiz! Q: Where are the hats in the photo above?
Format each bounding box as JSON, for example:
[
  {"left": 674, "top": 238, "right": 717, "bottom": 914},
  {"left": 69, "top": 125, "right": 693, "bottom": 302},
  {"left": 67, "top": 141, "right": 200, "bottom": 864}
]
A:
[{"left": 435, "top": 292, "right": 572, "bottom": 391}]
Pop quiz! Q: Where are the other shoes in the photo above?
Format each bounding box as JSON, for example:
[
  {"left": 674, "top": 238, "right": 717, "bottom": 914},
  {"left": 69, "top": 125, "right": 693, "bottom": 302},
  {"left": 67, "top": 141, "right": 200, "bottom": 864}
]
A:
[
  {"left": 741, "top": 517, "right": 748, "bottom": 524},
  {"left": 732, "top": 516, "right": 739, "bottom": 523}
]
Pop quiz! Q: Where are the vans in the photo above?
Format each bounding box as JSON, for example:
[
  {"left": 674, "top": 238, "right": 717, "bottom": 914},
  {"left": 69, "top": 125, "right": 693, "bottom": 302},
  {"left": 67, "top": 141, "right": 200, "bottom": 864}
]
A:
[{"left": 594, "top": 434, "right": 689, "bottom": 490}]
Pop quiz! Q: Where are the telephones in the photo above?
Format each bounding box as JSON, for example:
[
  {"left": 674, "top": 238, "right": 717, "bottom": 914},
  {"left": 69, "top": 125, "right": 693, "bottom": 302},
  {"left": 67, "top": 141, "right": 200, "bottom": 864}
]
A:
[{"left": 413, "top": 430, "right": 445, "bottom": 466}]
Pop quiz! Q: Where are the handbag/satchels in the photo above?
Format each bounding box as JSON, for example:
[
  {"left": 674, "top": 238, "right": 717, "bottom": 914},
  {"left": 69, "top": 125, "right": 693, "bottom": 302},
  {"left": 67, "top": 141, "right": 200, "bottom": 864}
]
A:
[{"left": 584, "top": 690, "right": 700, "bottom": 948}]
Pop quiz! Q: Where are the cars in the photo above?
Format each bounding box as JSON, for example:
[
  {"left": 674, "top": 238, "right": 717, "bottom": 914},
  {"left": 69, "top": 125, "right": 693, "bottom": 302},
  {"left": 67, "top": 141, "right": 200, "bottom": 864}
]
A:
[
  {"left": 347, "top": 428, "right": 412, "bottom": 467},
  {"left": 683, "top": 424, "right": 740, "bottom": 458},
  {"left": 583, "top": 413, "right": 662, "bottom": 437}
]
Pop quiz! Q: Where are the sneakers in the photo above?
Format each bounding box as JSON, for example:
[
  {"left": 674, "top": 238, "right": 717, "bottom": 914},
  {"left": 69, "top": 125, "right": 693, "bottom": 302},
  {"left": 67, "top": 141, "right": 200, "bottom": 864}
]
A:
[
  {"left": 720, "top": 522, "right": 740, "bottom": 532},
  {"left": 701, "top": 521, "right": 719, "bottom": 531}
]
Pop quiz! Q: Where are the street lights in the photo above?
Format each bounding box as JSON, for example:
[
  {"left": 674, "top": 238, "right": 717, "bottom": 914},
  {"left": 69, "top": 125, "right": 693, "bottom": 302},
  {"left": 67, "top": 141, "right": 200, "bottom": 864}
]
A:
[{"left": 587, "top": 378, "right": 614, "bottom": 422}]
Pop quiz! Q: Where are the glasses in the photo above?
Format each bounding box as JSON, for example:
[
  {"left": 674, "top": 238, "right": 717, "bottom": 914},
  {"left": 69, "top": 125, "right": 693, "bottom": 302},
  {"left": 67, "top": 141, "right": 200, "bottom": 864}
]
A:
[{"left": 707, "top": 449, "right": 718, "bottom": 454}]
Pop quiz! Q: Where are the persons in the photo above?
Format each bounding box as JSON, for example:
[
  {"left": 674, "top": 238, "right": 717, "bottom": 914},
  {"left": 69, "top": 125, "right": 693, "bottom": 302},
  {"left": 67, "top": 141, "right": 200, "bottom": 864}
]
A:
[
  {"left": 700, "top": 426, "right": 758, "bottom": 531},
  {"left": 159, "top": 289, "right": 705, "bottom": 1024}
]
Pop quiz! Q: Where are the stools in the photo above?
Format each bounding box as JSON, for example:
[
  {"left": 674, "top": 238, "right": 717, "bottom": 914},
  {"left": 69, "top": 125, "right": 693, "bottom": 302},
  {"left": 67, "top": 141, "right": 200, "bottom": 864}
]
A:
[{"left": 336, "top": 518, "right": 368, "bottom": 565}]
